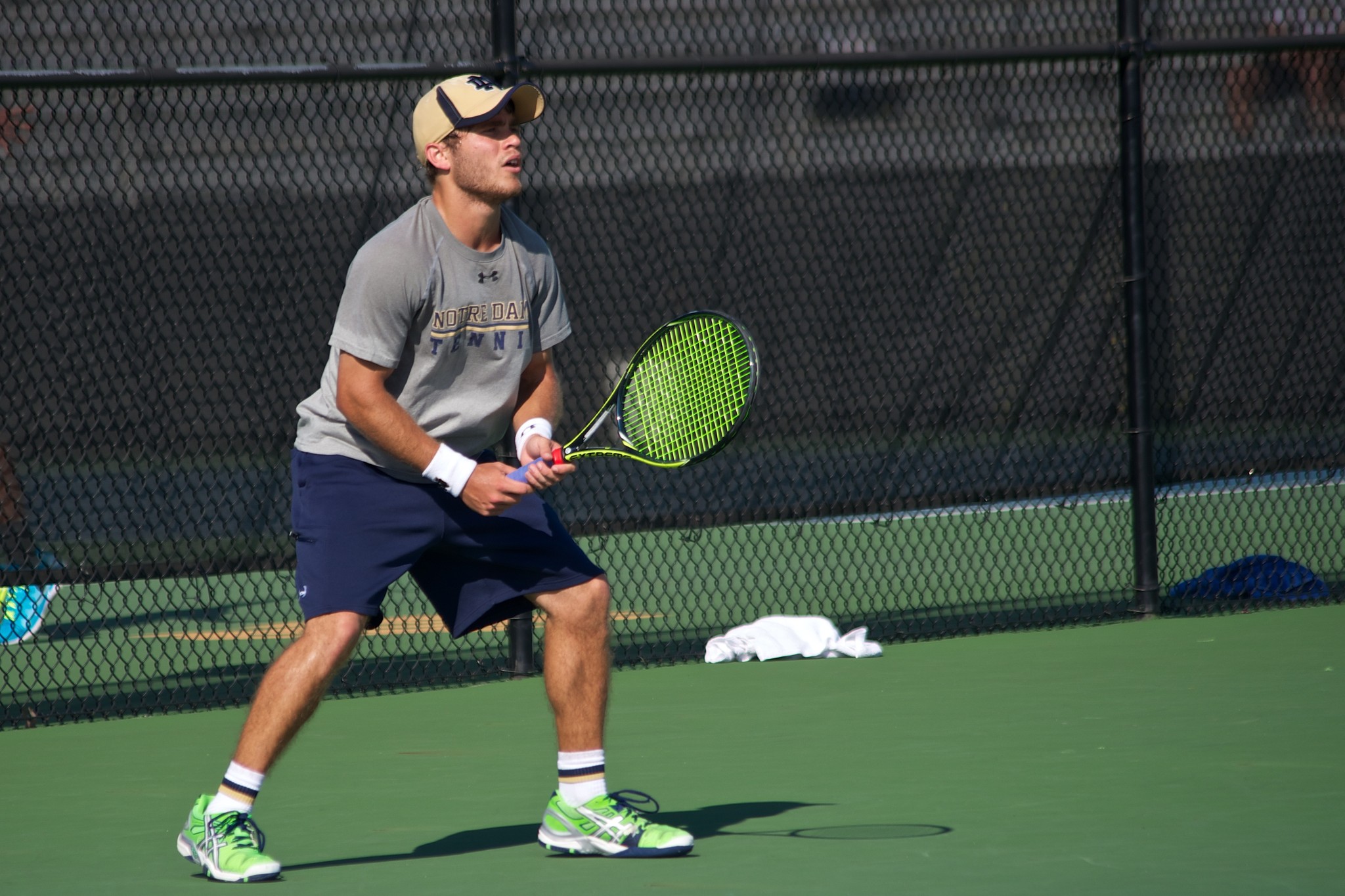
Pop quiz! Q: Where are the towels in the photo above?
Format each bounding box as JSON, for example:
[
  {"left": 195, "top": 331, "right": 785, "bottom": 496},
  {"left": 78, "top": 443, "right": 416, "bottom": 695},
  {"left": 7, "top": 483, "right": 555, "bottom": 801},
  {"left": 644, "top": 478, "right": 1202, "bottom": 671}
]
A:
[
  {"left": 703, "top": 610, "right": 884, "bottom": 664},
  {"left": 1167, "top": 555, "right": 1330, "bottom": 605}
]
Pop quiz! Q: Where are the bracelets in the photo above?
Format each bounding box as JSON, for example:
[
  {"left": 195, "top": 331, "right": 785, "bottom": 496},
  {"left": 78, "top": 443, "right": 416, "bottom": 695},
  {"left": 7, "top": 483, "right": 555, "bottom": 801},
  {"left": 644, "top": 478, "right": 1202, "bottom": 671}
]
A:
[
  {"left": 422, "top": 442, "right": 478, "bottom": 497},
  {"left": 514, "top": 418, "right": 552, "bottom": 460}
]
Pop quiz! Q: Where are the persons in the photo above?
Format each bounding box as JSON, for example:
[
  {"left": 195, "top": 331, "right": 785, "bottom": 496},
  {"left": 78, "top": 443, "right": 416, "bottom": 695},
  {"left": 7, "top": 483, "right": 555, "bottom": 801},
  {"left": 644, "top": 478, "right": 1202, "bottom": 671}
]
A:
[
  {"left": 0, "top": 445, "right": 60, "bottom": 645},
  {"left": 176, "top": 75, "right": 695, "bottom": 881}
]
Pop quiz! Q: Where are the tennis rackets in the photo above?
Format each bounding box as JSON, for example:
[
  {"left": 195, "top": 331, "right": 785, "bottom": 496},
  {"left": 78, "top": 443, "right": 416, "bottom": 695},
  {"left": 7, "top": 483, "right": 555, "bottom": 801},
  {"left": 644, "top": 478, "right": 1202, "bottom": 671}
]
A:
[{"left": 507, "top": 309, "right": 761, "bottom": 486}]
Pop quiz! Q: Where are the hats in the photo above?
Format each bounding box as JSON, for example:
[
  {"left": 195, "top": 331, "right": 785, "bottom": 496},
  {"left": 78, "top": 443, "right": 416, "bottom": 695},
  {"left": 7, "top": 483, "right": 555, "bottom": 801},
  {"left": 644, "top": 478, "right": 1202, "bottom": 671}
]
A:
[{"left": 412, "top": 73, "right": 546, "bottom": 167}]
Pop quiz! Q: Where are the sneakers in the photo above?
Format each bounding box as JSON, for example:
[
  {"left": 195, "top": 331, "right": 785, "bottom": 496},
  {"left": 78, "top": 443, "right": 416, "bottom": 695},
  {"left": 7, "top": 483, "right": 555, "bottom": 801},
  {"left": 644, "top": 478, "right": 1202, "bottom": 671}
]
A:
[
  {"left": 177, "top": 792, "right": 282, "bottom": 881},
  {"left": 538, "top": 789, "right": 694, "bottom": 857},
  {"left": 0, "top": 584, "right": 62, "bottom": 646}
]
